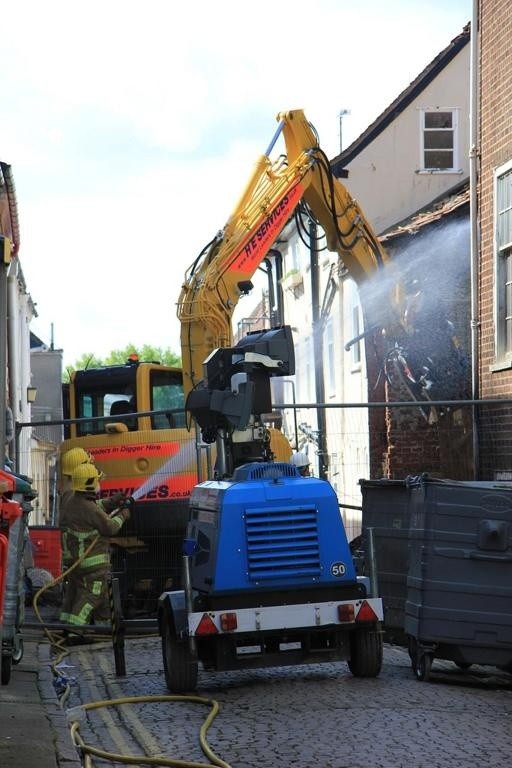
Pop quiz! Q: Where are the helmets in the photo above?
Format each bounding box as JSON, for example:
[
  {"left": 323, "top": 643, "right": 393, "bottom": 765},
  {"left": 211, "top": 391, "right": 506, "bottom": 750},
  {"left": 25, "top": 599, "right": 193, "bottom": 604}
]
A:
[
  {"left": 61, "top": 446, "right": 90, "bottom": 476},
  {"left": 70, "top": 463, "right": 98, "bottom": 492}
]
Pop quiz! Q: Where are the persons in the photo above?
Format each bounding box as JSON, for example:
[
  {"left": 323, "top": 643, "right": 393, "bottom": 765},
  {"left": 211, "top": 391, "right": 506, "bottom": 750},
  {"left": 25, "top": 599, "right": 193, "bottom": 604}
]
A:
[
  {"left": 56, "top": 446, "right": 115, "bottom": 628},
  {"left": 60, "top": 463, "right": 130, "bottom": 642}
]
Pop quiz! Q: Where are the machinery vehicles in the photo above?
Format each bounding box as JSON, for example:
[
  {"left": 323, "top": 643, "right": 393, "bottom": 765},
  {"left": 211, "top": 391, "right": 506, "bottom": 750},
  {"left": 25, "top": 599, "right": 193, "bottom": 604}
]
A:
[
  {"left": 156, "top": 322, "right": 390, "bottom": 694},
  {"left": 54, "top": 108, "right": 471, "bottom": 640}
]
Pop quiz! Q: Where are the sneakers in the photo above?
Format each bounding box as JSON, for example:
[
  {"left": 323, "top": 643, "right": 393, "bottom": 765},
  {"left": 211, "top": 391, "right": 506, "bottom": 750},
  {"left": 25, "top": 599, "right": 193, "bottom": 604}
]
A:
[{"left": 66, "top": 636, "right": 93, "bottom": 646}]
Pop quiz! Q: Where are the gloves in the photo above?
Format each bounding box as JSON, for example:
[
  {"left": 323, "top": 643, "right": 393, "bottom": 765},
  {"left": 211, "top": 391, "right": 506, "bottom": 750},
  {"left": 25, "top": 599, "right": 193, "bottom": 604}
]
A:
[
  {"left": 117, "top": 509, "right": 133, "bottom": 523},
  {"left": 104, "top": 493, "right": 129, "bottom": 512}
]
